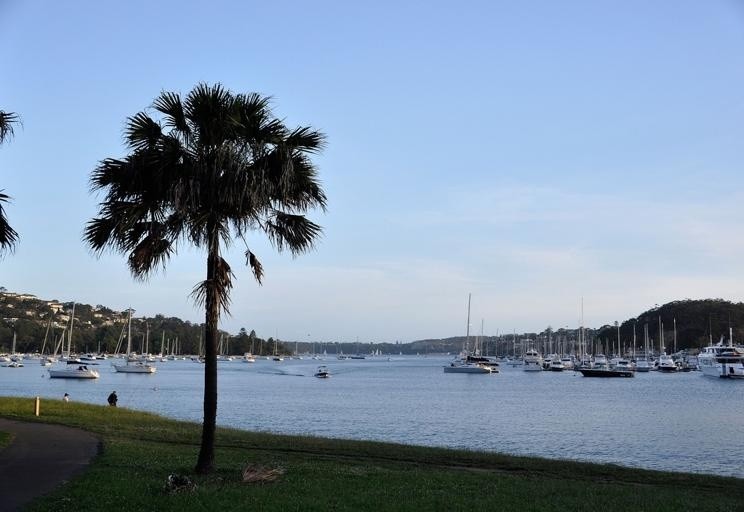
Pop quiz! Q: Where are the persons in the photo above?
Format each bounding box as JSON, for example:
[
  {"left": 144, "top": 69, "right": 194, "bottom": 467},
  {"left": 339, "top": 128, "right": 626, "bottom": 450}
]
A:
[
  {"left": 107, "top": 391, "right": 117, "bottom": 406},
  {"left": 63, "top": 392, "right": 70, "bottom": 401}
]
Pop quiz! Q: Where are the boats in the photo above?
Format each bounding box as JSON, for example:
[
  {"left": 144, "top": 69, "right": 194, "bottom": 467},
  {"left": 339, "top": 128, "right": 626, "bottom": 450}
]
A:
[{"left": 314, "top": 366, "right": 330, "bottom": 379}]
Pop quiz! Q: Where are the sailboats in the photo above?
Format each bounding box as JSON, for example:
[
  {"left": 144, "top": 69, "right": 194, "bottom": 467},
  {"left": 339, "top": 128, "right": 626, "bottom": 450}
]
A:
[
  {"left": 242, "top": 335, "right": 365, "bottom": 363},
  {"left": 444, "top": 293, "right": 744, "bottom": 380},
  {"left": 0, "top": 298, "right": 236, "bottom": 379}
]
